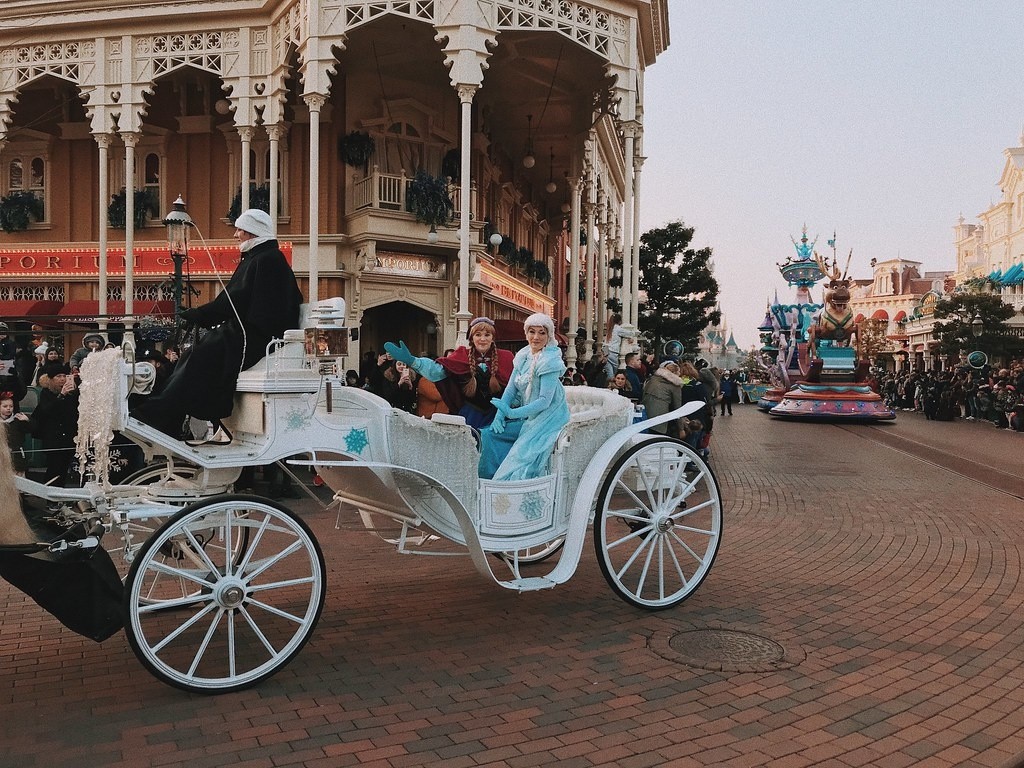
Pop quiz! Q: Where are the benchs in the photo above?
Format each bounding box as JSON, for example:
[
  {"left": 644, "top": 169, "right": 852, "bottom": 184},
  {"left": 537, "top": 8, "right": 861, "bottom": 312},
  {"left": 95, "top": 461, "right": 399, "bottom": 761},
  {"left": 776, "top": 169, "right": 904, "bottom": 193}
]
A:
[
  {"left": 550, "top": 384, "right": 634, "bottom": 469},
  {"left": 233, "top": 297, "right": 352, "bottom": 395}
]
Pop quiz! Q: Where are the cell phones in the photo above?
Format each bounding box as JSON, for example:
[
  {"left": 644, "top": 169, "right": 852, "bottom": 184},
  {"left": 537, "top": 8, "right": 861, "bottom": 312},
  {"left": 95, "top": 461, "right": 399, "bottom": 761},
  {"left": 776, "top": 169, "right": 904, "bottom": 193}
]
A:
[
  {"left": 65, "top": 375, "right": 74, "bottom": 390},
  {"left": 601, "top": 345, "right": 609, "bottom": 356},
  {"left": 403, "top": 369, "right": 409, "bottom": 377}
]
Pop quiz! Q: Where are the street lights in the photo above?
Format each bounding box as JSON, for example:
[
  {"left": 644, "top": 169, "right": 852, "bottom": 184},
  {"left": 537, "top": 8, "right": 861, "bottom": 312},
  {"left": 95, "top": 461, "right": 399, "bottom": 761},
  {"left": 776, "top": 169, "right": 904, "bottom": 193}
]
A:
[{"left": 159, "top": 194, "right": 194, "bottom": 340}]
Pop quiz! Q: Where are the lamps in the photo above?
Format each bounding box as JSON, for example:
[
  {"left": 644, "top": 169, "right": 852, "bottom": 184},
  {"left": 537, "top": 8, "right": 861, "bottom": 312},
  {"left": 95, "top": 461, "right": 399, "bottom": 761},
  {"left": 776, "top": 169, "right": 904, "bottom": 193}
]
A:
[
  {"left": 490, "top": 220, "right": 502, "bottom": 244},
  {"left": 215, "top": 99, "right": 230, "bottom": 116},
  {"left": 560, "top": 172, "right": 570, "bottom": 212},
  {"left": 426, "top": 322, "right": 437, "bottom": 341},
  {"left": 427, "top": 224, "right": 439, "bottom": 243},
  {"left": 524, "top": 114, "right": 535, "bottom": 167},
  {"left": 547, "top": 147, "right": 557, "bottom": 192}
]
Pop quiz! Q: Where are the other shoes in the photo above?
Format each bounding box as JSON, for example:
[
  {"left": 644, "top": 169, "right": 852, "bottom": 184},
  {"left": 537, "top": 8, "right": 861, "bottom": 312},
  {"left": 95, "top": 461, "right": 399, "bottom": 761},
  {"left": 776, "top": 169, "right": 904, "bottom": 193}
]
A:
[
  {"left": 728, "top": 411, "right": 733, "bottom": 416},
  {"left": 721, "top": 413, "right": 725, "bottom": 416}
]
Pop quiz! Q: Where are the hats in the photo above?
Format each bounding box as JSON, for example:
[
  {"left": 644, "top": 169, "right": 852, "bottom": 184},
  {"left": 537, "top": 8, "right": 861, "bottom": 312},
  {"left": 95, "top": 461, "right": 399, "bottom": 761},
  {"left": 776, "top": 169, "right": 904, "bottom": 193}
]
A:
[
  {"left": 0, "top": 322, "right": 9, "bottom": 331},
  {"left": 136, "top": 347, "right": 161, "bottom": 361},
  {"left": 34, "top": 342, "right": 49, "bottom": 354},
  {"left": 681, "top": 417, "right": 690, "bottom": 426},
  {"left": 688, "top": 420, "right": 703, "bottom": 432},
  {"left": 48, "top": 364, "right": 69, "bottom": 379},
  {"left": 85, "top": 337, "right": 102, "bottom": 347},
  {"left": 235, "top": 208, "right": 273, "bottom": 236},
  {"left": 466, "top": 316, "right": 496, "bottom": 339}
]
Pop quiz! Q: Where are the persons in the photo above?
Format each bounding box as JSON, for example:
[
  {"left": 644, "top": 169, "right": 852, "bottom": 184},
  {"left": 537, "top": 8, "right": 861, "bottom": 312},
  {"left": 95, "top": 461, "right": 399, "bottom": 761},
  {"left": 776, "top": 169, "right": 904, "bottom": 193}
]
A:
[
  {"left": 384, "top": 317, "right": 515, "bottom": 429},
  {"left": 0, "top": 313, "right": 1024, "bottom": 471},
  {"left": 129, "top": 209, "right": 303, "bottom": 441},
  {"left": 479, "top": 313, "right": 571, "bottom": 481}
]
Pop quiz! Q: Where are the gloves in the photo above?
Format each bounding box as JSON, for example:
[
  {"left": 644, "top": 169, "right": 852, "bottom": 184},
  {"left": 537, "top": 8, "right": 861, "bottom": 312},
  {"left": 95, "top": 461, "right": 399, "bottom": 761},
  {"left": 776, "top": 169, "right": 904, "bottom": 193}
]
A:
[
  {"left": 489, "top": 416, "right": 506, "bottom": 434},
  {"left": 384, "top": 340, "right": 415, "bottom": 367},
  {"left": 177, "top": 306, "right": 207, "bottom": 328},
  {"left": 490, "top": 397, "right": 512, "bottom": 419}
]
